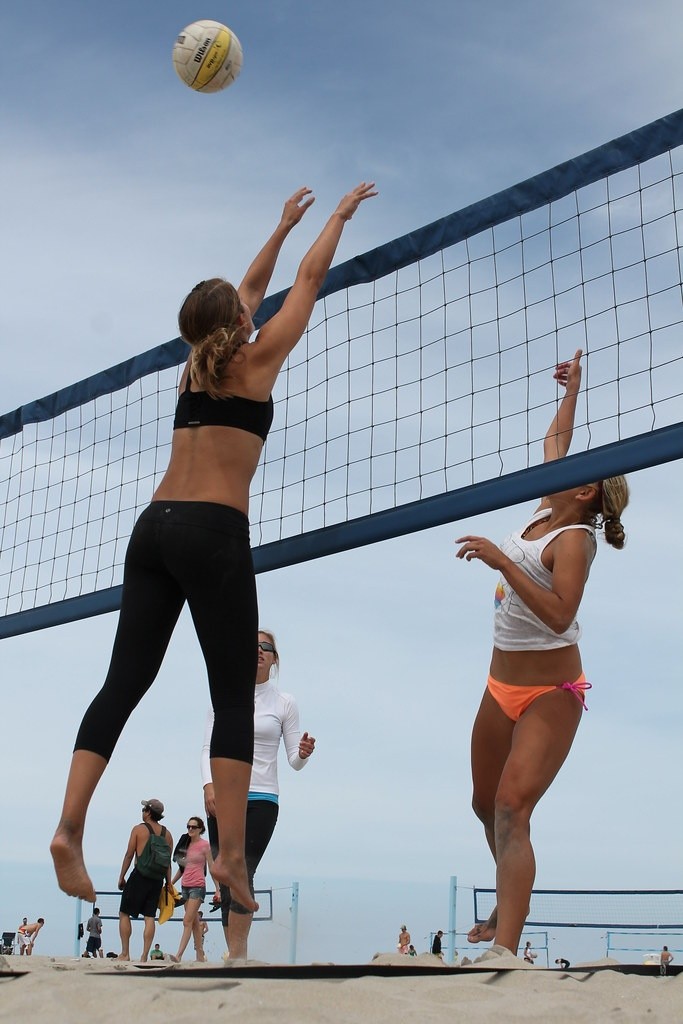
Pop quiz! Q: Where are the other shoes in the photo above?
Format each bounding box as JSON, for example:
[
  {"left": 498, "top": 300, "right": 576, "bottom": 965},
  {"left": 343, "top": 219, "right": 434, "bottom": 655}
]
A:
[
  {"left": 208, "top": 896, "right": 223, "bottom": 905},
  {"left": 209, "top": 902, "right": 222, "bottom": 913}
]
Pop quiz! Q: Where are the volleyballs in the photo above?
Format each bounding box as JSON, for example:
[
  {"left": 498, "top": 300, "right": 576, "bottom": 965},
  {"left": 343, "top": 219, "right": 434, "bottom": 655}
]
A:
[{"left": 174, "top": 20, "right": 242, "bottom": 92}]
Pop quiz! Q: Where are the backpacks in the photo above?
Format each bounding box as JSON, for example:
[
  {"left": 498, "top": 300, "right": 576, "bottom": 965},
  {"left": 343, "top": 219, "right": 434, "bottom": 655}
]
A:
[{"left": 136, "top": 823, "right": 171, "bottom": 884}]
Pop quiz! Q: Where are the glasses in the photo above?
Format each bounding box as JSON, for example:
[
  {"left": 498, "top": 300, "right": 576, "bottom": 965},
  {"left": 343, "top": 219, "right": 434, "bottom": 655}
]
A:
[
  {"left": 187, "top": 825, "right": 200, "bottom": 829},
  {"left": 258, "top": 642, "right": 276, "bottom": 654}
]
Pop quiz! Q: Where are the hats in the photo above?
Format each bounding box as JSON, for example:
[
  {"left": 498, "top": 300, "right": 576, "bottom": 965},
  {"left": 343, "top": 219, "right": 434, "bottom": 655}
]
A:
[{"left": 141, "top": 799, "right": 164, "bottom": 813}]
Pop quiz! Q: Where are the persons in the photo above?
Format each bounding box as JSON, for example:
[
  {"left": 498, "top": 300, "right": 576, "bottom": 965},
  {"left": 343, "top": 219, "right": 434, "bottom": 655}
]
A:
[
  {"left": 114, "top": 799, "right": 173, "bottom": 962},
  {"left": 150, "top": 943, "right": 164, "bottom": 960},
  {"left": 555, "top": 959, "right": 570, "bottom": 968},
  {"left": 398, "top": 925, "right": 411, "bottom": 954},
  {"left": 659, "top": 946, "right": 674, "bottom": 976},
  {"left": 408, "top": 945, "right": 417, "bottom": 957},
  {"left": 169, "top": 817, "right": 221, "bottom": 964},
  {"left": 524, "top": 942, "right": 532, "bottom": 963},
  {"left": 86, "top": 908, "right": 103, "bottom": 958},
  {"left": 202, "top": 630, "right": 316, "bottom": 957},
  {"left": 432, "top": 930, "right": 444, "bottom": 959},
  {"left": 18, "top": 918, "right": 45, "bottom": 955},
  {"left": 194, "top": 911, "right": 209, "bottom": 956},
  {"left": 454, "top": 349, "right": 629, "bottom": 956},
  {"left": 50, "top": 181, "right": 378, "bottom": 912}
]
